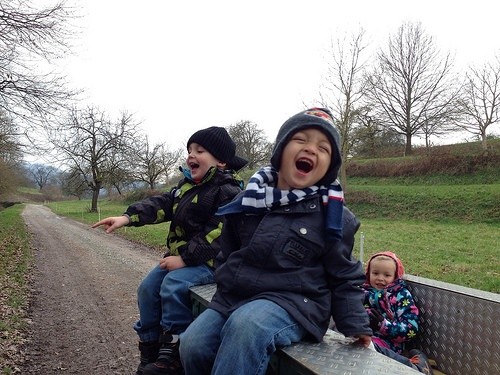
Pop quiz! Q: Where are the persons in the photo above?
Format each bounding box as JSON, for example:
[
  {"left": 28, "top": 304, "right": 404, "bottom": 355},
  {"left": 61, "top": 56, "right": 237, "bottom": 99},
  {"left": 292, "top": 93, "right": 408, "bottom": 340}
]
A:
[
  {"left": 179, "top": 108, "right": 372, "bottom": 375},
  {"left": 92, "top": 126, "right": 248, "bottom": 375},
  {"left": 362, "top": 251, "right": 434, "bottom": 375}
]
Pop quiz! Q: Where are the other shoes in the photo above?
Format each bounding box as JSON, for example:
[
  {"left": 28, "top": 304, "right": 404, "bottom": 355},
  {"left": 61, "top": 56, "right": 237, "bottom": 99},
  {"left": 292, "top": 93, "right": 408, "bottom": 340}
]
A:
[
  {"left": 409, "top": 349, "right": 433, "bottom": 375},
  {"left": 135, "top": 341, "right": 173, "bottom": 373}
]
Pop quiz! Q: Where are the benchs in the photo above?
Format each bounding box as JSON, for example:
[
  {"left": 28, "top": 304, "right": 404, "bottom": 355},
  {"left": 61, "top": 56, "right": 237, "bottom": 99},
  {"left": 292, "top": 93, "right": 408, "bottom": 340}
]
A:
[
  {"left": 404, "top": 273, "right": 500, "bottom": 375},
  {"left": 191, "top": 275, "right": 431, "bottom": 375}
]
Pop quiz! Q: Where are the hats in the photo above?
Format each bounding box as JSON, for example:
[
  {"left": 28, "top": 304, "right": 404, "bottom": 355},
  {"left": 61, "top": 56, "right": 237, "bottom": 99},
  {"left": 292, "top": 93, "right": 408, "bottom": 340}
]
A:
[
  {"left": 365, "top": 252, "right": 403, "bottom": 286},
  {"left": 271, "top": 107, "right": 342, "bottom": 185},
  {"left": 186, "top": 126, "right": 248, "bottom": 170}
]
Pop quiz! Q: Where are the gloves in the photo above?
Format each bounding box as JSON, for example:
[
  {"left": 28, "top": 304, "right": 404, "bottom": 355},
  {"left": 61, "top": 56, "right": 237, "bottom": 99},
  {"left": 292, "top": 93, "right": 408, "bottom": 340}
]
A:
[{"left": 368, "top": 309, "right": 386, "bottom": 331}]
